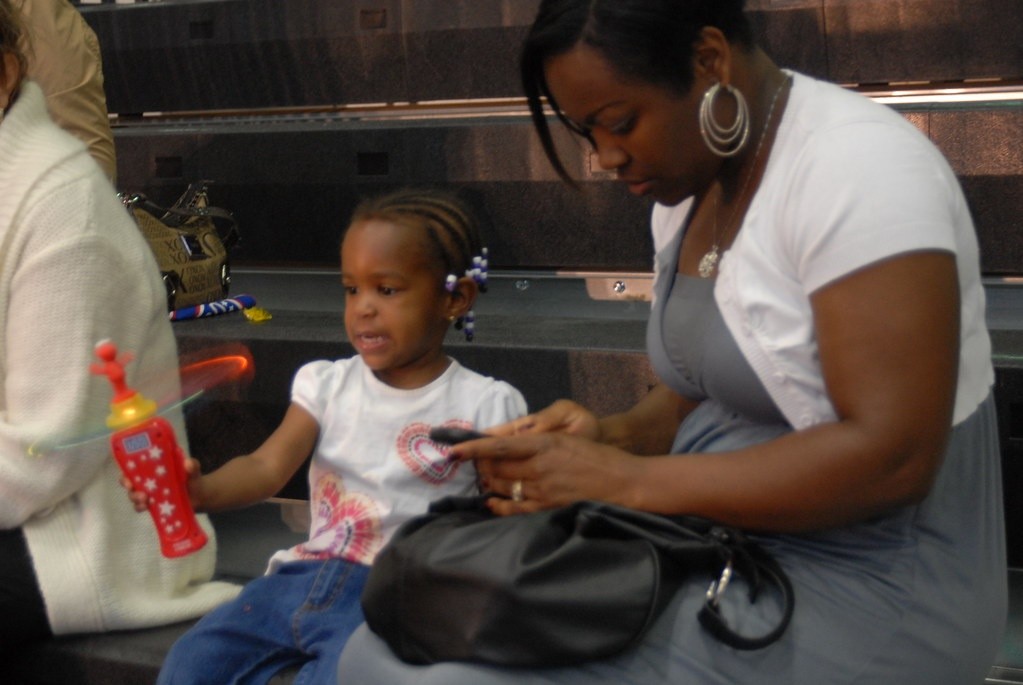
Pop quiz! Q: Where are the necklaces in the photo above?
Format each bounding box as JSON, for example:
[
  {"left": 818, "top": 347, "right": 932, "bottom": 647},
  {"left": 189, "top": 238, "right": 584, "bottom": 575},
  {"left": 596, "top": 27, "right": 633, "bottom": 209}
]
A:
[{"left": 699, "top": 76, "right": 790, "bottom": 277}]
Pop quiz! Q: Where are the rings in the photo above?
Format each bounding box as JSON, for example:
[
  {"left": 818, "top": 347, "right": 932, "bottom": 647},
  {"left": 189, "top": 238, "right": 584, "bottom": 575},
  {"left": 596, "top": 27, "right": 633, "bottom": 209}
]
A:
[{"left": 511, "top": 479, "right": 525, "bottom": 501}]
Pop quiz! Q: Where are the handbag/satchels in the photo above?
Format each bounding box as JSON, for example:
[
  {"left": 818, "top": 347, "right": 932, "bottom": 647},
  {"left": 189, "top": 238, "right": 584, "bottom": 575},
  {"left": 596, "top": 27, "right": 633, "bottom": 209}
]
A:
[
  {"left": 359, "top": 490, "right": 795, "bottom": 670},
  {"left": 116, "top": 176, "right": 236, "bottom": 308}
]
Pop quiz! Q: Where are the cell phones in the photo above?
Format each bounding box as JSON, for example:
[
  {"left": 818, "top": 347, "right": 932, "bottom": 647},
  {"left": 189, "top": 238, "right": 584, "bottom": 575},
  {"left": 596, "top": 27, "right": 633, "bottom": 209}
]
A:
[{"left": 428, "top": 427, "right": 490, "bottom": 446}]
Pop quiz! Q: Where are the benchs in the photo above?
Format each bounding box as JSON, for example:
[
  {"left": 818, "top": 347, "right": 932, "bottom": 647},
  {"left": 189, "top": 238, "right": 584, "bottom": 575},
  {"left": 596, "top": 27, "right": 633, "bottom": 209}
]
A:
[{"left": 56, "top": 269, "right": 1023, "bottom": 685}]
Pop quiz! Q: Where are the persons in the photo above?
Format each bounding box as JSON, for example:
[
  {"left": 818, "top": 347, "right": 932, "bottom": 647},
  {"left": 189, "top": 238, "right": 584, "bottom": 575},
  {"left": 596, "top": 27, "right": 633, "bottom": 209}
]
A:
[
  {"left": 5, "top": 1, "right": 115, "bottom": 196},
  {"left": 0, "top": 0, "right": 218, "bottom": 684},
  {"left": 118, "top": 184, "right": 527, "bottom": 682},
  {"left": 337, "top": 1, "right": 1007, "bottom": 685}
]
